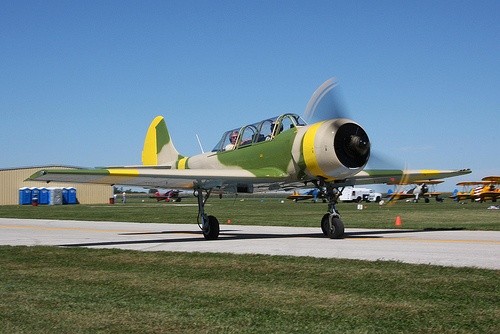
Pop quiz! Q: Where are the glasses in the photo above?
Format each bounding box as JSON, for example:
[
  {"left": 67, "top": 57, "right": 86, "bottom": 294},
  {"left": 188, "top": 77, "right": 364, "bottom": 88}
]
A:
[{"left": 232, "top": 136, "right": 240, "bottom": 139}]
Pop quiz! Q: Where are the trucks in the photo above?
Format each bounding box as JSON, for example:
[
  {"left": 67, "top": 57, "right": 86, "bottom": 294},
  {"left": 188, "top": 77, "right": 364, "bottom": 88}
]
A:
[{"left": 339, "top": 187, "right": 372, "bottom": 202}]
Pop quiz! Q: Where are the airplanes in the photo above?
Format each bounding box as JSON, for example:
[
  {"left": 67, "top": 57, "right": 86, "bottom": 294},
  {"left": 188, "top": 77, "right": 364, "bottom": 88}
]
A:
[
  {"left": 23, "top": 77, "right": 472, "bottom": 240},
  {"left": 449, "top": 176, "right": 500, "bottom": 203},
  {"left": 387, "top": 183, "right": 441, "bottom": 202}
]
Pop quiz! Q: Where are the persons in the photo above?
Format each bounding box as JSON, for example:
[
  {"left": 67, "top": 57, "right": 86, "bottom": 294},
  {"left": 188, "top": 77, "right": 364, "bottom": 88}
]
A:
[
  {"left": 224, "top": 131, "right": 239, "bottom": 150},
  {"left": 265, "top": 121, "right": 283, "bottom": 142},
  {"left": 122, "top": 191, "right": 126, "bottom": 204}
]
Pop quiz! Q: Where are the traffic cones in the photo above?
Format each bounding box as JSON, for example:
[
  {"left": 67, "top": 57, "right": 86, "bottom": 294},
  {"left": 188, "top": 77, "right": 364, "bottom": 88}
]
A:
[{"left": 394, "top": 215, "right": 402, "bottom": 225}]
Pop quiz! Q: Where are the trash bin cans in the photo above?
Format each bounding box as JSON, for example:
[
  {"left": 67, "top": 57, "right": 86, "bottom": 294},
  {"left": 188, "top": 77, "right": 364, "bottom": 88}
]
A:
[
  {"left": 19, "top": 187, "right": 39, "bottom": 205},
  {"left": 39, "top": 187, "right": 61, "bottom": 205},
  {"left": 62, "top": 187, "right": 76, "bottom": 204}
]
